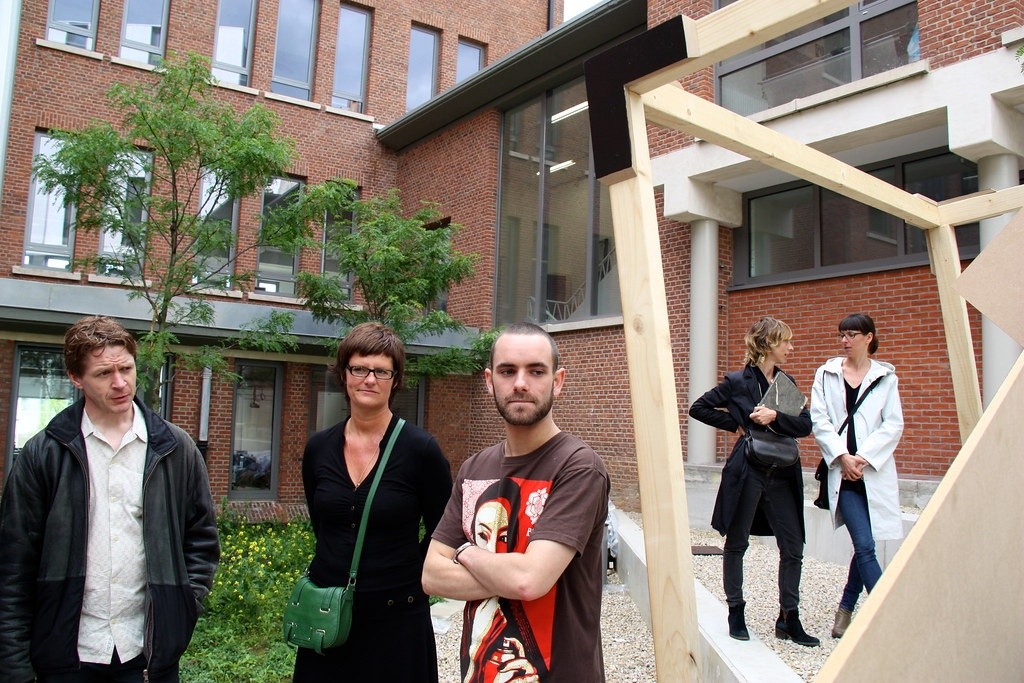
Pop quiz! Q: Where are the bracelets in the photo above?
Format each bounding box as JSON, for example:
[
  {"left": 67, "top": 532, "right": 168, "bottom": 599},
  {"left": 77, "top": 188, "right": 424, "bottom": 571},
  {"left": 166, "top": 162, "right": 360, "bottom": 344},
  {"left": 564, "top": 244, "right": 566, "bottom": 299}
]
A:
[{"left": 453, "top": 542, "right": 472, "bottom": 563}]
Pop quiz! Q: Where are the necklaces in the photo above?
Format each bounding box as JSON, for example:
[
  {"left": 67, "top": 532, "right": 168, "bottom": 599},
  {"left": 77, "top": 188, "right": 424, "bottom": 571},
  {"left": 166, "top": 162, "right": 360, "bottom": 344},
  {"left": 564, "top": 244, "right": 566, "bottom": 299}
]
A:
[{"left": 349, "top": 426, "right": 379, "bottom": 487}]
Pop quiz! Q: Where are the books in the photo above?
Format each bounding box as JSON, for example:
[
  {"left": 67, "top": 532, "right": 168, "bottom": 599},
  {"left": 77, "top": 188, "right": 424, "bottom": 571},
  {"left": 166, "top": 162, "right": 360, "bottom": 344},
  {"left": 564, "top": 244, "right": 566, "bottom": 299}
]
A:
[{"left": 753, "top": 372, "right": 808, "bottom": 433}]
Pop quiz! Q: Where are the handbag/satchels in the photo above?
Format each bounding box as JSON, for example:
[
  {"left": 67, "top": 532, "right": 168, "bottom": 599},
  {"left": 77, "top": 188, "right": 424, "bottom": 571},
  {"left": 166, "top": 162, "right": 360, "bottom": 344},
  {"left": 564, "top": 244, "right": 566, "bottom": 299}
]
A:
[
  {"left": 283, "top": 576, "right": 353, "bottom": 655},
  {"left": 744, "top": 427, "right": 799, "bottom": 470},
  {"left": 813, "top": 457, "right": 829, "bottom": 510}
]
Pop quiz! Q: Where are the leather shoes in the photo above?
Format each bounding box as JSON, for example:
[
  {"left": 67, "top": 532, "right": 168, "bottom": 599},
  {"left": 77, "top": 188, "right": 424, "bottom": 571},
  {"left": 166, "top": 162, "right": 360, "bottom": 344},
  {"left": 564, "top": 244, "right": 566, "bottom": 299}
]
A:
[{"left": 832, "top": 607, "right": 851, "bottom": 636}]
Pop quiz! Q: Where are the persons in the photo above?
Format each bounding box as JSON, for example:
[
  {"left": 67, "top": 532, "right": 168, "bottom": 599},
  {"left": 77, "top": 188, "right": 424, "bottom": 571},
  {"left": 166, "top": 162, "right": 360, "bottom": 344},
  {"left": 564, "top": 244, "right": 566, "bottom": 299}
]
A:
[
  {"left": 421, "top": 322, "right": 612, "bottom": 683},
  {"left": 0, "top": 316, "right": 220, "bottom": 682},
  {"left": 293, "top": 322, "right": 454, "bottom": 683},
  {"left": 810, "top": 313, "right": 904, "bottom": 638},
  {"left": 689, "top": 318, "right": 819, "bottom": 646}
]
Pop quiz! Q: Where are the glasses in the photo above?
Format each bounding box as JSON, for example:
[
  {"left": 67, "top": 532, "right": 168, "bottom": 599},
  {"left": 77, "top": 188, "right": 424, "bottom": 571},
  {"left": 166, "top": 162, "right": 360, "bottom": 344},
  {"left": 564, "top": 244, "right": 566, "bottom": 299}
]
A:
[
  {"left": 837, "top": 332, "right": 866, "bottom": 339},
  {"left": 345, "top": 363, "right": 398, "bottom": 380}
]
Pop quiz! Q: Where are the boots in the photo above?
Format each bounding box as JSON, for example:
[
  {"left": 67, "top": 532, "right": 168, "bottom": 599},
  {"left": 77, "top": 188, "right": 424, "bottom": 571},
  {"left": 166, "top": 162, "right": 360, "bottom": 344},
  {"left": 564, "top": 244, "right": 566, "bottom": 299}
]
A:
[
  {"left": 727, "top": 600, "right": 750, "bottom": 641},
  {"left": 776, "top": 605, "right": 819, "bottom": 646}
]
